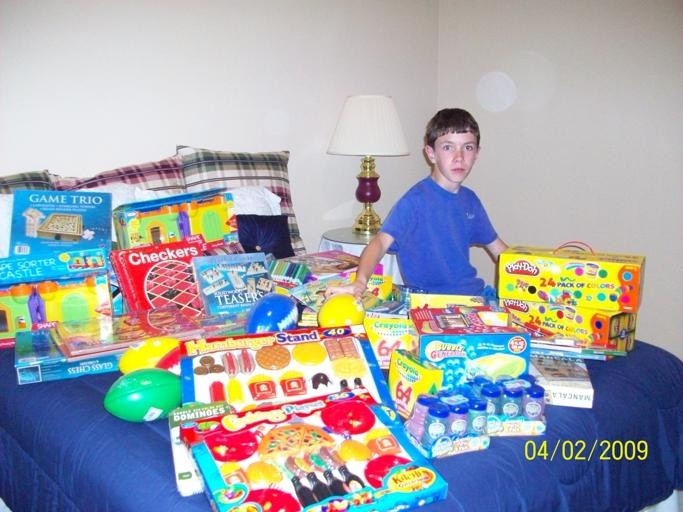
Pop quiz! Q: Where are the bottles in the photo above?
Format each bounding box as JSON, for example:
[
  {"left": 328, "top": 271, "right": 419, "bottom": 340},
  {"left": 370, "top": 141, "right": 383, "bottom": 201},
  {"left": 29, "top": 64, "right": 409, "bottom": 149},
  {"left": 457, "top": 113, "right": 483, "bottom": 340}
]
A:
[
  {"left": 408, "top": 373, "right": 544, "bottom": 450},
  {"left": 352, "top": 377, "right": 367, "bottom": 392},
  {"left": 339, "top": 380, "right": 350, "bottom": 392}
]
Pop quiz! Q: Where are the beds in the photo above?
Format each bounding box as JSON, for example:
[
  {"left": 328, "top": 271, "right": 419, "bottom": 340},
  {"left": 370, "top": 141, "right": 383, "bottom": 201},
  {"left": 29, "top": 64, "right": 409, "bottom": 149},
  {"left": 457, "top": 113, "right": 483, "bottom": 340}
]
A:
[{"left": 0, "top": 264, "right": 682, "bottom": 512}]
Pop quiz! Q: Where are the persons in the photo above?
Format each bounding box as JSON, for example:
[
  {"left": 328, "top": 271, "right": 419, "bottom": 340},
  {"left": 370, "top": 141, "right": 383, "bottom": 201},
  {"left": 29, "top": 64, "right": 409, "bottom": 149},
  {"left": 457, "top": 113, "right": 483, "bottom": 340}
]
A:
[{"left": 324, "top": 108, "right": 510, "bottom": 303}]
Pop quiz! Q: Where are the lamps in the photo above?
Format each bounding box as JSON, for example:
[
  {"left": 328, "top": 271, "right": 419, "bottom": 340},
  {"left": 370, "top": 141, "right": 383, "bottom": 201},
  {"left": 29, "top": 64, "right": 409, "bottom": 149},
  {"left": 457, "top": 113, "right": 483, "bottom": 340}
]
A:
[{"left": 328, "top": 92, "right": 409, "bottom": 234}]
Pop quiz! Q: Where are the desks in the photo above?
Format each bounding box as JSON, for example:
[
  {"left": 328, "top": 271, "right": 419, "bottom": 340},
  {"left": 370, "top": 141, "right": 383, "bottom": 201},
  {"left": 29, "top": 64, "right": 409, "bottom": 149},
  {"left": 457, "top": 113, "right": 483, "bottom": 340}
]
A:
[{"left": 314, "top": 224, "right": 402, "bottom": 285}]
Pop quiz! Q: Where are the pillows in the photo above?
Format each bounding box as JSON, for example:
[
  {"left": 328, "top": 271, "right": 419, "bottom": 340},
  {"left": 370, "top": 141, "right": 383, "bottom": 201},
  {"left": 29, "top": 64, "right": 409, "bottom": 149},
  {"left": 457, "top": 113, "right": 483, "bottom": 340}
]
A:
[
  {"left": 0, "top": 181, "right": 133, "bottom": 259},
  {"left": 174, "top": 143, "right": 307, "bottom": 254},
  {"left": 235, "top": 213, "right": 295, "bottom": 259},
  {"left": 59, "top": 157, "right": 186, "bottom": 198},
  {"left": 133, "top": 182, "right": 282, "bottom": 215},
  {"left": 1, "top": 166, "right": 53, "bottom": 193}
]
